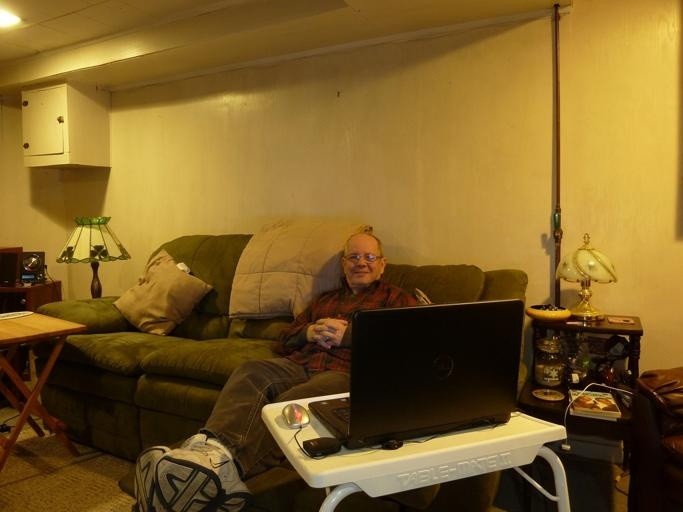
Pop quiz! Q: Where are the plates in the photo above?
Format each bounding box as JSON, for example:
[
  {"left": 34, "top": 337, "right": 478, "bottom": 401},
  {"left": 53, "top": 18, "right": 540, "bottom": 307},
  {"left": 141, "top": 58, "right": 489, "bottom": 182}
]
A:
[{"left": 0, "top": 311, "right": 33, "bottom": 321}]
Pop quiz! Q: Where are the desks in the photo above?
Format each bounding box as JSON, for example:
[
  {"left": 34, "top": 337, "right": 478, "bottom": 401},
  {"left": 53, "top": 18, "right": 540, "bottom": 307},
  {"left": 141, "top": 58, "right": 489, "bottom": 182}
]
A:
[
  {"left": 0, "top": 309, "right": 87, "bottom": 471},
  {"left": 259, "top": 384, "right": 574, "bottom": 511}
]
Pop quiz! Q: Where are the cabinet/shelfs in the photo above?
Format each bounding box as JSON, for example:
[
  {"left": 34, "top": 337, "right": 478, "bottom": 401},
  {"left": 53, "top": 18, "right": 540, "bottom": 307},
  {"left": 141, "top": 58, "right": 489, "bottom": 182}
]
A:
[
  {"left": 0, "top": 280, "right": 62, "bottom": 381},
  {"left": 21, "top": 82, "right": 111, "bottom": 169},
  {"left": 517, "top": 314, "right": 642, "bottom": 512}
]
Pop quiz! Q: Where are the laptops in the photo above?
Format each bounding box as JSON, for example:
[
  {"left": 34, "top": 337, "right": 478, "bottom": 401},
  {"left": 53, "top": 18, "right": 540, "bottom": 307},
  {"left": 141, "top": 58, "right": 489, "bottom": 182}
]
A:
[{"left": 308, "top": 299, "right": 525, "bottom": 449}]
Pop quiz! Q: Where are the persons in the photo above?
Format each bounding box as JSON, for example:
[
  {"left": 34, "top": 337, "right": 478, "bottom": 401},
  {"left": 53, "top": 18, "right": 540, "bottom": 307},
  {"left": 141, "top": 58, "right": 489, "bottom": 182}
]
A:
[{"left": 134, "top": 229, "right": 420, "bottom": 512}]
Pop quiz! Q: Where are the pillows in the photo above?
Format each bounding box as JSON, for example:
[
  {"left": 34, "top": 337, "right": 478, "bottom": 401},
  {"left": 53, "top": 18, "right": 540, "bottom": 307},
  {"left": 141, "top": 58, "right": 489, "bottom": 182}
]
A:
[{"left": 112, "top": 249, "right": 214, "bottom": 338}]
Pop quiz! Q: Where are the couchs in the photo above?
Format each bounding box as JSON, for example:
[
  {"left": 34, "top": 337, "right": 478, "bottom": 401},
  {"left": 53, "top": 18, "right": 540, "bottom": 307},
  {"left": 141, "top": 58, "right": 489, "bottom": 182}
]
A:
[
  {"left": 33, "top": 232, "right": 529, "bottom": 511},
  {"left": 635, "top": 364, "right": 683, "bottom": 512}
]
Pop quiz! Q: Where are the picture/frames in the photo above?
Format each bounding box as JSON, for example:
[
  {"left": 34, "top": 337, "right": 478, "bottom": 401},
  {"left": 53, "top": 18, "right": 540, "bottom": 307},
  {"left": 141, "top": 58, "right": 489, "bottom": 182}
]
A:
[{"left": 0, "top": 246, "right": 22, "bottom": 288}]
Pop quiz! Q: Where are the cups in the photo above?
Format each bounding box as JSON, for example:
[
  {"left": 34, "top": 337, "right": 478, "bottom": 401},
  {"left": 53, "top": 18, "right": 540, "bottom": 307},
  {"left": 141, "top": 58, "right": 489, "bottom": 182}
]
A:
[{"left": 323, "top": 319, "right": 348, "bottom": 347}]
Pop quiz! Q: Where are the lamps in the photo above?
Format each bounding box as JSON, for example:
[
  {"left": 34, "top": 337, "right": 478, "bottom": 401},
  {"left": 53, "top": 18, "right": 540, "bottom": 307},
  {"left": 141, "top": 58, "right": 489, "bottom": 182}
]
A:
[
  {"left": 55, "top": 216, "right": 130, "bottom": 299},
  {"left": 555, "top": 233, "right": 618, "bottom": 323}
]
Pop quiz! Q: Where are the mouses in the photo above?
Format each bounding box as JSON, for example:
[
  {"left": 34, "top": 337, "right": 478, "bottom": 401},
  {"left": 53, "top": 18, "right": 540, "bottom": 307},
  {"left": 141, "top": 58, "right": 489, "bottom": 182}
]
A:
[{"left": 282, "top": 403, "right": 310, "bottom": 429}]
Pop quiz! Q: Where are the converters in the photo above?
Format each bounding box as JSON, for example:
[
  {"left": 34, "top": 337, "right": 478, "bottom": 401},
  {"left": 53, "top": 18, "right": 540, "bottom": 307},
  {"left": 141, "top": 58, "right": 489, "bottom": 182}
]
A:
[{"left": 303, "top": 437, "right": 341, "bottom": 456}]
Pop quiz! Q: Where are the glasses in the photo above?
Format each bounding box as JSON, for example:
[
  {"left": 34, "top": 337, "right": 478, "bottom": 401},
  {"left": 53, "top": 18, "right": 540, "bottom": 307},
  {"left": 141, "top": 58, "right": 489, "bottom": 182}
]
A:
[{"left": 347, "top": 255, "right": 382, "bottom": 262}]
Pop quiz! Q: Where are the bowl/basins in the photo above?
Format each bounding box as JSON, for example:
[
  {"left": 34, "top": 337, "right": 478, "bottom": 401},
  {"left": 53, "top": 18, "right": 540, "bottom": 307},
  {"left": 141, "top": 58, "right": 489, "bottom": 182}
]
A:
[{"left": 525, "top": 304, "right": 572, "bottom": 322}]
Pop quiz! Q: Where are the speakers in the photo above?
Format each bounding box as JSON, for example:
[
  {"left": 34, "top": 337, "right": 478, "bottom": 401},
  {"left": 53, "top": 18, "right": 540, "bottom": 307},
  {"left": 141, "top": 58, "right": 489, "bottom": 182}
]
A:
[{"left": 20, "top": 252, "right": 45, "bottom": 283}]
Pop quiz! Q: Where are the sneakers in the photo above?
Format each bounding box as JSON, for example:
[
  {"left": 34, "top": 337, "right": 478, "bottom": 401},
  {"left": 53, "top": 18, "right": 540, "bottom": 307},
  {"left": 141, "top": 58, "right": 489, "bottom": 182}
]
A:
[
  {"left": 133, "top": 446, "right": 171, "bottom": 512},
  {"left": 156, "top": 434, "right": 253, "bottom": 512}
]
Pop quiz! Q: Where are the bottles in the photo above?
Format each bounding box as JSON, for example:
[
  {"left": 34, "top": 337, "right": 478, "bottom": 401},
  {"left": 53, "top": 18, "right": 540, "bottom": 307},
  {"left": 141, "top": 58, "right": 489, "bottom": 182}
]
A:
[{"left": 534, "top": 328, "right": 589, "bottom": 388}]
